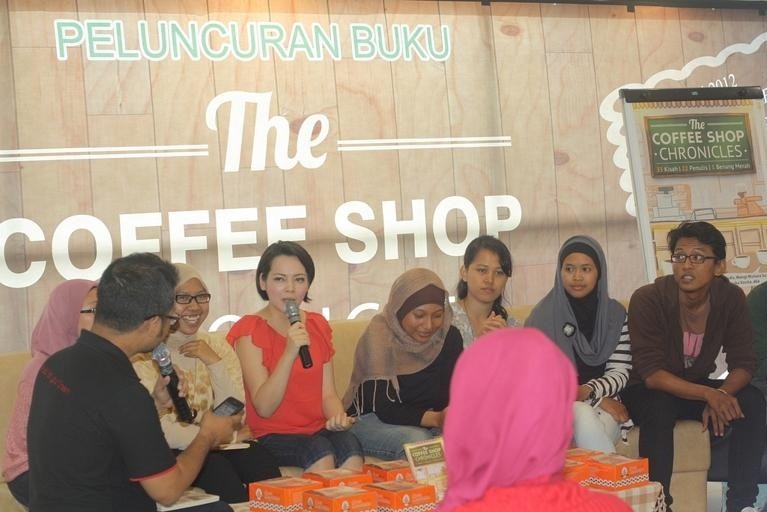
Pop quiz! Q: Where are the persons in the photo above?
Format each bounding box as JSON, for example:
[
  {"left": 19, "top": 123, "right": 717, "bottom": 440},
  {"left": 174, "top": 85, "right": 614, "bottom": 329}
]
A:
[
  {"left": 433, "top": 323, "right": 633, "bottom": 512},
  {"left": 24, "top": 250, "right": 245, "bottom": 511},
  {"left": 0, "top": 276, "right": 101, "bottom": 512},
  {"left": 450, "top": 234, "right": 525, "bottom": 351},
  {"left": 339, "top": 267, "right": 464, "bottom": 465},
  {"left": 127, "top": 261, "right": 281, "bottom": 503},
  {"left": 523, "top": 234, "right": 635, "bottom": 457},
  {"left": 225, "top": 238, "right": 366, "bottom": 475},
  {"left": 616, "top": 218, "right": 754, "bottom": 512},
  {"left": 726, "top": 278, "right": 767, "bottom": 512}
]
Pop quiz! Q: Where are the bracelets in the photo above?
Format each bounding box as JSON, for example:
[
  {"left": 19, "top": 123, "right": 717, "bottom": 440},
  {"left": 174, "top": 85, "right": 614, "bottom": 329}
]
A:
[{"left": 716, "top": 387, "right": 729, "bottom": 397}]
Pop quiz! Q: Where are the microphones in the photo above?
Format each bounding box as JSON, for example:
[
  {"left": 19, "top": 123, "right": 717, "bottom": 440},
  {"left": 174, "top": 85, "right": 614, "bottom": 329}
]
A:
[
  {"left": 152, "top": 342, "right": 192, "bottom": 423},
  {"left": 286, "top": 299, "right": 314, "bottom": 370}
]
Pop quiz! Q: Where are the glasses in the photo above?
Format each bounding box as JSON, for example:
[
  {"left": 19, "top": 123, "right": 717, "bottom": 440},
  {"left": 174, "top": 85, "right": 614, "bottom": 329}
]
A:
[
  {"left": 670, "top": 254, "right": 721, "bottom": 264},
  {"left": 175, "top": 293, "right": 211, "bottom": 304},
  {"left": 144, "top": 311, "right": 180, "bottom": 325}
]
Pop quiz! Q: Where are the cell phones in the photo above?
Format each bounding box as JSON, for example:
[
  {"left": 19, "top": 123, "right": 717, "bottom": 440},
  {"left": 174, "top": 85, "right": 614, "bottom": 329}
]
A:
[{"left": 214, "top": 395, "right": 245, "bottom": 417}]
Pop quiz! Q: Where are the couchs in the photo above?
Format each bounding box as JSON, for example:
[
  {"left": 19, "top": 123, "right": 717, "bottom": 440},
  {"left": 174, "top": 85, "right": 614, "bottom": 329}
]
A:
[{"left": 0, "top": 300, "right": 712, "bottom": 512}]
